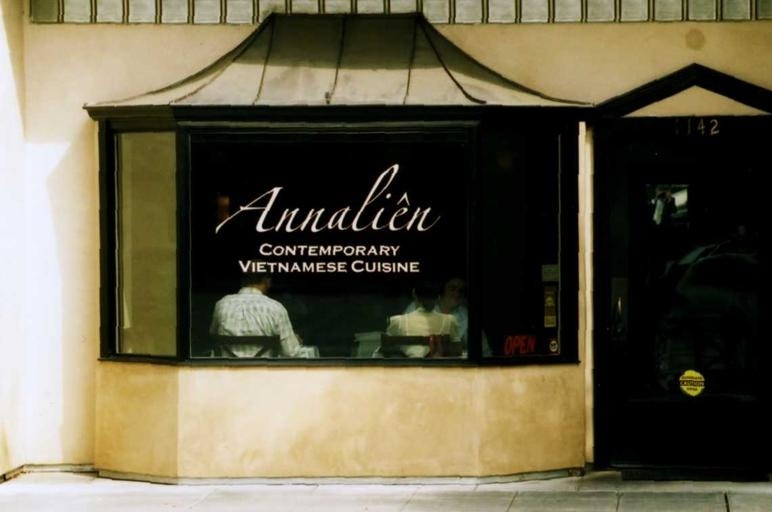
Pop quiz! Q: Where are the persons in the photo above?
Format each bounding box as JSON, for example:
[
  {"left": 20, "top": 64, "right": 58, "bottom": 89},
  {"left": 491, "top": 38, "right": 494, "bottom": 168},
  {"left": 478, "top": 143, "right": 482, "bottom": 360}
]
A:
[
  {"left": 371, "top": 269, "right": 471, "bottom": 360},
  {"left": 208, "top": 258, "right": 321, "bottom": 360}
]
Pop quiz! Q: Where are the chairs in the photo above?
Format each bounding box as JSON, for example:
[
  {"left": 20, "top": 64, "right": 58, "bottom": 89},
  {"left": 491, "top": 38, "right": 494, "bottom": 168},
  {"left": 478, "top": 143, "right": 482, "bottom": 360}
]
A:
[
  {"left": 212, "top": 333, "right": 280, "bottom": 357},
  {"left": 381, "top": 334, "right": 449, "bottom": 357}
]
